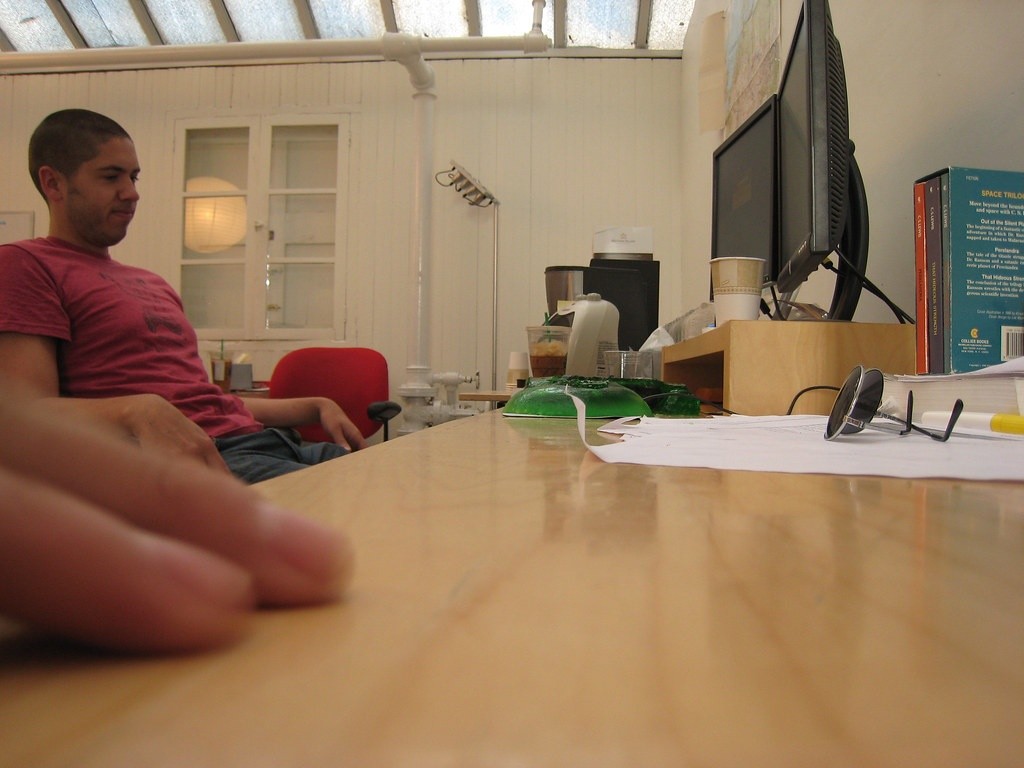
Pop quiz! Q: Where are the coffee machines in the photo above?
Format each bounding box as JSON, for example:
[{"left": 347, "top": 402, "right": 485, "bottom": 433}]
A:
[{"left": 545, "top": 258, "right": 661, "bottom": 349}]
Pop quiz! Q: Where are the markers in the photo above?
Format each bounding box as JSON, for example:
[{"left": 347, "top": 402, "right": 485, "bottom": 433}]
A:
[{"left": 922, "top": 411, "right": 1024, "bottom": 435}]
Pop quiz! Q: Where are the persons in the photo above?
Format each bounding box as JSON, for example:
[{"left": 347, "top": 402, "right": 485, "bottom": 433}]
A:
[
  {"left": 0, "top": 108, "right": 369, "bottom": 486},
  {"left": 0, "top": 379, "right": 354, "bottom": 651}
]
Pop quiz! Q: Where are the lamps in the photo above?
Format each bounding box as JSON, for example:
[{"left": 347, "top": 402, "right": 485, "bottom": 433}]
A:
[{"left": 184, "top": 177, "right": 247, "bottom": 254}]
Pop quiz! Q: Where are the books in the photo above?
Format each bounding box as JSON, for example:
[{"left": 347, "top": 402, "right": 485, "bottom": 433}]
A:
[{"left": 913, "top": 166, "right": 1024, "bottom": 374}]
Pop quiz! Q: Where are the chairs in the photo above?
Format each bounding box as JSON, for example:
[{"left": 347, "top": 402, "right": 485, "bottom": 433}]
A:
[{"left": 266, "top": 346, "right": 403, "bottom": 443}]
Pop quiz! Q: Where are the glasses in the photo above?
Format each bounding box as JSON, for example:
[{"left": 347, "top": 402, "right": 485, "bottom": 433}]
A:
[{"left": 823, "top": 365, "right": 963, "bottom": 442}]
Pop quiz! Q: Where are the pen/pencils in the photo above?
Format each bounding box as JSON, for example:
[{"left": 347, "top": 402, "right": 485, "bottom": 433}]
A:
[{"left": 220, "top": 339, "right": 224, "bottom": 361}]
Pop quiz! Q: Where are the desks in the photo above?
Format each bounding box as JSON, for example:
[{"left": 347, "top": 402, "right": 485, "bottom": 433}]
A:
[
  {"left": 458, "top": 389, "right": 511, "bottom": 401},
  {"left": 0, "top": 409, "right": 1024, "bottom": 768}
]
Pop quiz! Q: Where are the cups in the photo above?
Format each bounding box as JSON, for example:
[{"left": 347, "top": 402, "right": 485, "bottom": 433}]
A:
[
  {"left": 209, "top": 351, "right": 232, "bottom": 392},
  {"left": 526, "top": 325, "right": 571, "bottom": 375},
  {"left": 621, "top": 352, "right": 653, "bottom": 379},
  {"left": 506, "top": 351, "right": 530, "bottom": 393},
  {"left": 604, "top": 351, "right": 638, "bottom": 378},
  {"left": 709, "top": 257, "right": 766, "bottom": 330}
]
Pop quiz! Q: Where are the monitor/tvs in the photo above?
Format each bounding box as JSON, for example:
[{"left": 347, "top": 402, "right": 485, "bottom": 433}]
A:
[{"left": 706, "top": 0, "right": 869, "bottom": 319}]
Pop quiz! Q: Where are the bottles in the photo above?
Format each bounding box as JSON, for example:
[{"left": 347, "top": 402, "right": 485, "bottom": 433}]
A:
[
  {"left": 566, "top": 293, "right": 618, "bottom": 376},
  {"left": 512, "top": 379, "right": 526, "bottom": 397}
]
